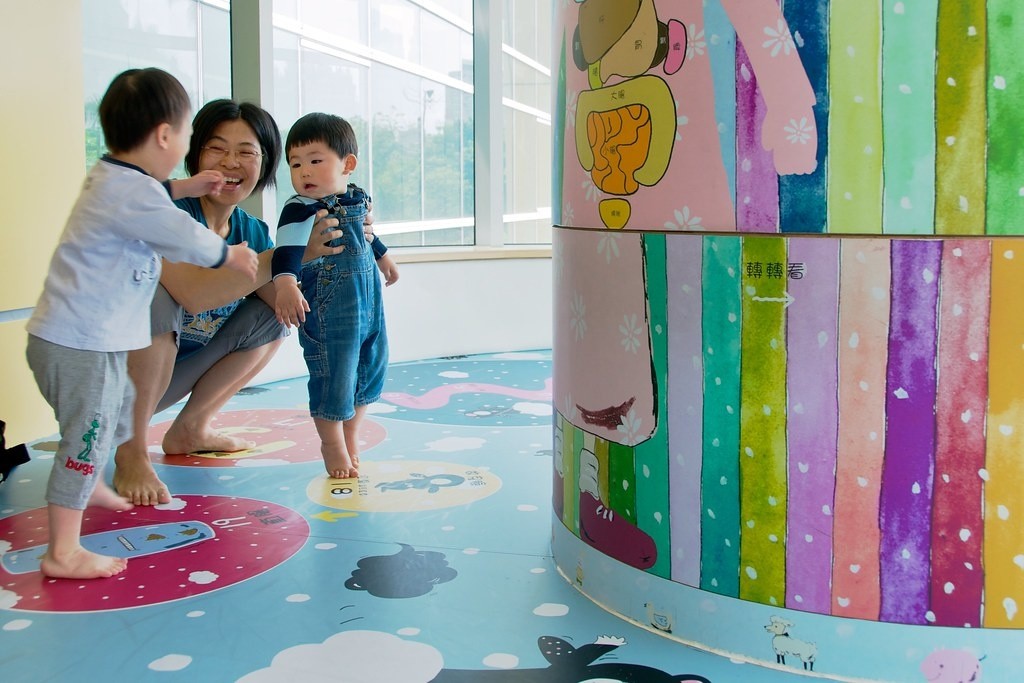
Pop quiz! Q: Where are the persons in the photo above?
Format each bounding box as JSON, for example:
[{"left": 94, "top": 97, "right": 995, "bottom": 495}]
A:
[
  {"left": 113, "top": 98, "right": 282, "bottom": 506},
  {"left": 25, "top": 67, "right": 259, "bottom": 578},
  {"left": 271, "top": 112, "right": 398, "bottom": 478}
]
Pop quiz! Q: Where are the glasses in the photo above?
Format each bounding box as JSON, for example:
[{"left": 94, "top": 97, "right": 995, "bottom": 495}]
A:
[{"left": 202, "top": 144, "right": 265, "bottom": 162}]
